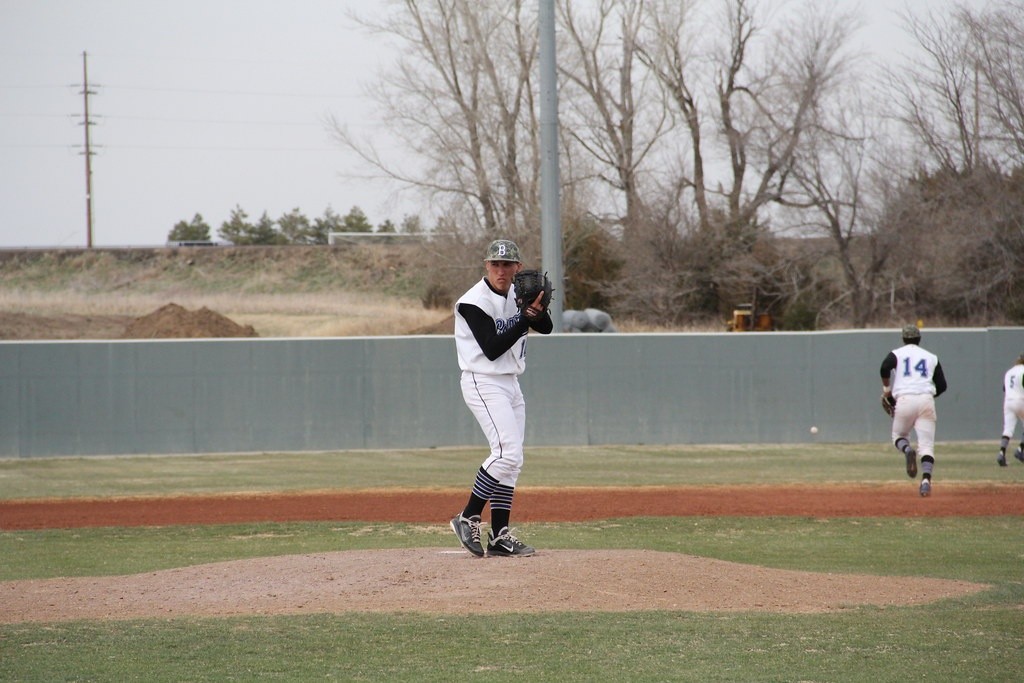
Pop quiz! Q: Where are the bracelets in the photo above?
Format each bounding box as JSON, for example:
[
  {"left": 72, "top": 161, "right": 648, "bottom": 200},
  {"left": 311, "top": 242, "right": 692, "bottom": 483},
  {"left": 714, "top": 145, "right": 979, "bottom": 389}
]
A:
[{"left": 882, "top": 386, "right": 891, "bottom": 393}]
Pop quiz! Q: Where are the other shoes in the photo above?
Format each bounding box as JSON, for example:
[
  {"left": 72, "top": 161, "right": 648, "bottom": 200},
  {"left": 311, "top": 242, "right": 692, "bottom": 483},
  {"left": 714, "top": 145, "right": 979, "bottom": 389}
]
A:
[
  {"left": 1014, "top": 449, "right": 1024, "bottom": 463},
  {"left": 906, "top": 449, "right": 918, "bottom": 478},
  {"left": 997, "top": 454, "right": 1007, "bottom": 466},
  {"left": 920, "top": 482, "right": 931, "bottom": 496}
]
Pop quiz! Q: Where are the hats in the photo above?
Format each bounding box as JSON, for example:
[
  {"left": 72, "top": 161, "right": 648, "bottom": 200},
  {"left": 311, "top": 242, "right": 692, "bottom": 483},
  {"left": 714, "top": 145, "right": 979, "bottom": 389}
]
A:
[
  {"left": 483, "top": 240, "right": 520, "bottom": 264},
  {"left": 902, "top": 324, "right": 921, "bottom": 338}
]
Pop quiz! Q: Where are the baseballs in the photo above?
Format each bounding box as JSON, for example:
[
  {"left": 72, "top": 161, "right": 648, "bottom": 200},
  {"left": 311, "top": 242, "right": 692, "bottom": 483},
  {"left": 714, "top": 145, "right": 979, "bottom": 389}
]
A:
[{"left": 810, "top": 426, "right": 819, "bottom": 434}]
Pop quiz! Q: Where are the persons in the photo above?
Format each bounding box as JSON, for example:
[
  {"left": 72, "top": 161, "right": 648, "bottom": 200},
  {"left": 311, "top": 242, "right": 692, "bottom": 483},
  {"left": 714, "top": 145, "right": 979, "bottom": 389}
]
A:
[
  {"left": 450, "top": 239, "right": 553, "bottom": 557},
  {"left": 880, "top": 325, "right": 947, "bottom": 497},
  {"left": 997, "top": 352, "right": 1024, "bottom": 467}
]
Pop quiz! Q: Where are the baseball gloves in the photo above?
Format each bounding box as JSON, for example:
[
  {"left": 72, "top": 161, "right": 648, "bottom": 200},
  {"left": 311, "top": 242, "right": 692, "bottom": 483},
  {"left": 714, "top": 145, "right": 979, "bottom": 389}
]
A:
[
  {"left": 882, "top": 396, "right": 897, "bottom": 417},
  {"left": 514, "top": 269, "right": 552, "bottom": 311}
]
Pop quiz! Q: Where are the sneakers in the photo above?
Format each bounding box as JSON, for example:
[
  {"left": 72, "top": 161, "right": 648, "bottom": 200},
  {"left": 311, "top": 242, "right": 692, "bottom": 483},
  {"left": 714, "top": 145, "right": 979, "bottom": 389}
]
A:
[
  {"left": 487, "top": 526, "right": 535, "bottom": 557},
  {"left": 450, "top": 511, "right": 484, "bottom": 558}
]
